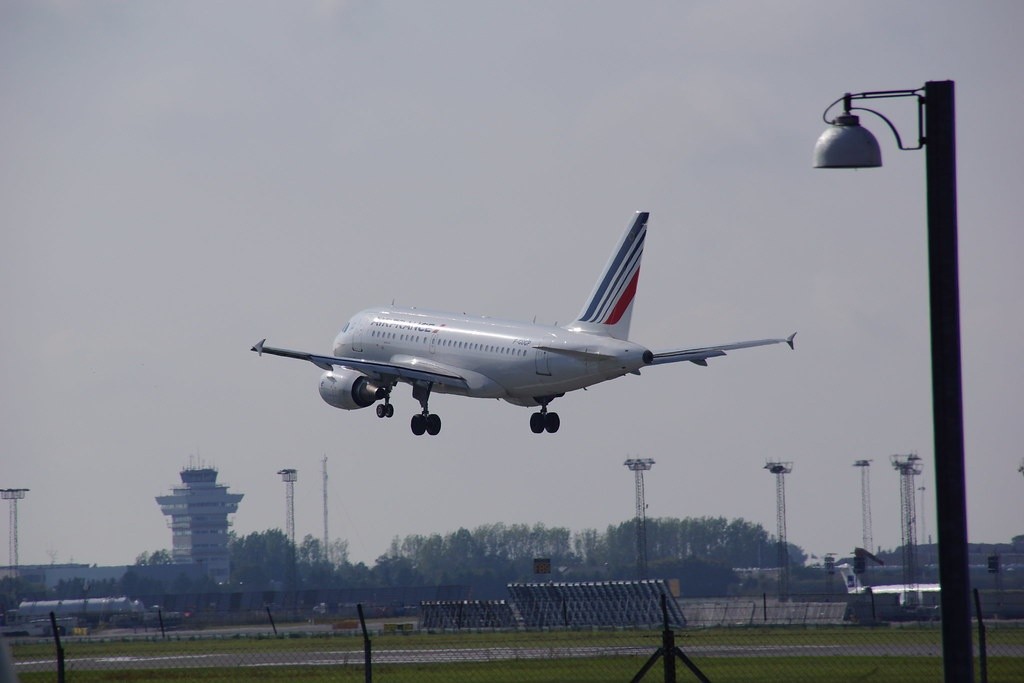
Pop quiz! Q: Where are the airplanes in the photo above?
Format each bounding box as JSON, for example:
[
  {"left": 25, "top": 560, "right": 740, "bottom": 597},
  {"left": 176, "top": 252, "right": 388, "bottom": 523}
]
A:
[{"left": 248, "top": 210, "right": 801, "bottom": 436}]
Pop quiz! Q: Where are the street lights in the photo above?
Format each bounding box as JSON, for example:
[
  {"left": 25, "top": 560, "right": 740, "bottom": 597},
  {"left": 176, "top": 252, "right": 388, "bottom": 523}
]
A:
[{"left": 811, "top": 80, "right": 976, "bottom": 683}]
